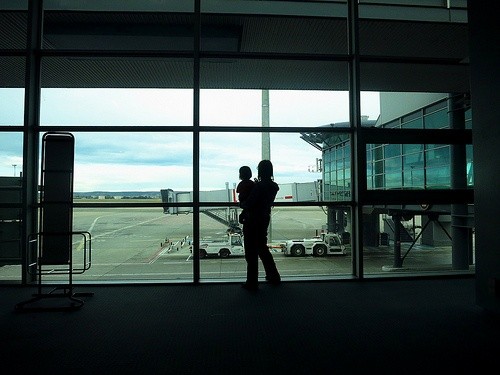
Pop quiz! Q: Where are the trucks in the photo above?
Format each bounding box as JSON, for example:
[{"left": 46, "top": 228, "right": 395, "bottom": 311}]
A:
[
  {"left": 283, "top": 231, "right": 344, "bottom": 257},
  {"left": 188, "top": 233, "right": 243, "bottom": 258}
]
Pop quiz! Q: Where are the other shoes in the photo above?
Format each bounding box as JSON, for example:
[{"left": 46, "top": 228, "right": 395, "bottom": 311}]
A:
[
  {"left": 266, "top": 277, "right": 281, "bottom": 284},
  {"left": 242, "top": 281, "right": 260, "bottom": 291}
]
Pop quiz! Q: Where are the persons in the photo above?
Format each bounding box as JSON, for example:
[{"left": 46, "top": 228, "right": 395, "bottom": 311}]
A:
[
  {"left": 241, "top": 159, "right": 281, "bottom": 291},
  {"left": 235, "top": 166, "right": 258, "bottom": 223}
]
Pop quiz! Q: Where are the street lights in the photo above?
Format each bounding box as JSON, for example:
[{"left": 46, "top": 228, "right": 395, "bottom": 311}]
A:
[{"left": 12, "top": 165, "right": 17, "bottom": 177}]
